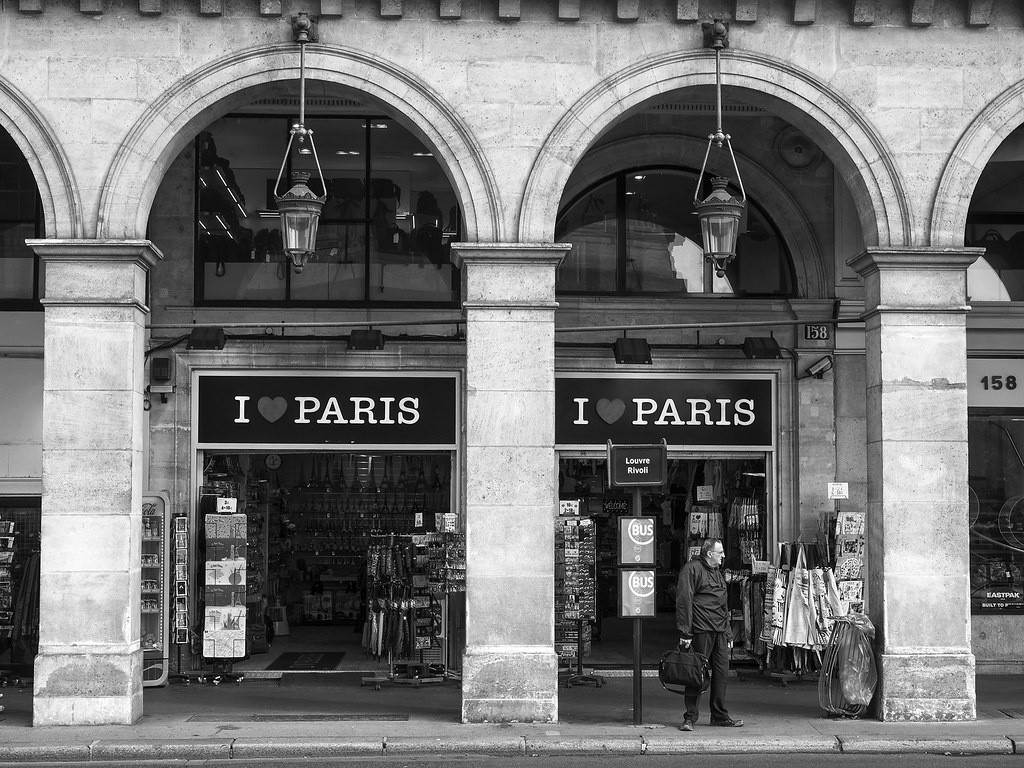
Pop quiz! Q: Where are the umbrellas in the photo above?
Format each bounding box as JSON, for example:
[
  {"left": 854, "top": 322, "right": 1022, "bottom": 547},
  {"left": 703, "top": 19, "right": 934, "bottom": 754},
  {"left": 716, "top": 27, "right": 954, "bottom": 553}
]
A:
[{"left": 363, "top": 545, "right": 414, "bottom": 662}]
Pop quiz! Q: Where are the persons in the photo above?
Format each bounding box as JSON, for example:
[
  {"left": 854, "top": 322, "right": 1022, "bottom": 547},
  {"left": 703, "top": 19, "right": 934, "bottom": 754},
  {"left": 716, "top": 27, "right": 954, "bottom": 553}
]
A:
[{"left": 675, "top": 538, "right": 743, "bottom": 730}]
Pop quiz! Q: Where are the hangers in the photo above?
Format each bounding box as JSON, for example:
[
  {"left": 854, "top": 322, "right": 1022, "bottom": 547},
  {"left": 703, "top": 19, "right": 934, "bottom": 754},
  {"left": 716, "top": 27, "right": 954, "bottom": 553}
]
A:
[{"left": 730, "top": 489, "right": 762, "bottom": 538}]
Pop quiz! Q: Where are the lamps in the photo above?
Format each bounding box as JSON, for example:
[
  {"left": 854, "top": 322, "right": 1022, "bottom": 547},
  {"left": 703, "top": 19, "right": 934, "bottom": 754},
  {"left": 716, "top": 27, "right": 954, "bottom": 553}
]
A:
[
  {"left": 345, "top": 328, "right": 387, "bottom": 351},
  {"left": 691, "top": 18, "right": 748, "bottom": 280},
  {"left": 611, "top": 337, "right": 651, "bottom": 364},
  {"left": 738, "top": 337, "right": 784, "bottom": 359},
  {"left": 186, "top": 325, "right": 228, "bottom": 354},
  {"left": 275, "top": 11, "right": 328, "bottom": 275}
]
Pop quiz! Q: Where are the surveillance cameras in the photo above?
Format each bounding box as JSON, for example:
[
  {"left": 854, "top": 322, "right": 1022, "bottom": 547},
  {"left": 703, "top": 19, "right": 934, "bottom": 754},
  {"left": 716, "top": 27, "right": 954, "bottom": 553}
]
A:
[
  {"left": 805, "top": 355, "right": 833, "bottom": 376},
  {"left": 149, "top": 385, "right": 174, "bottom": 393}
]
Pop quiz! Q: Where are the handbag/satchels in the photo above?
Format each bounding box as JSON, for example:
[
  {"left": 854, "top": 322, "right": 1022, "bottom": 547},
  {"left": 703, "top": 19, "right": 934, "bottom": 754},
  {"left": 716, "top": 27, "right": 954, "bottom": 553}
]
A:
[
  {"left": 659, "top": 644, "right": 713, "bottom": 695},
  {"left": 745, "top": 540, "right": 846, "bottom": 675}
]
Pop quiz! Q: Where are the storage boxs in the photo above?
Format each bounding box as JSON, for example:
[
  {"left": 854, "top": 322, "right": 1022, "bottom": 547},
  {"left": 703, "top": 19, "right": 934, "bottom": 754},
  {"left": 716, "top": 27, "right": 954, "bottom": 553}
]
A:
[{"left": 265, "top": 175, "right": 398, "bottom": 218}]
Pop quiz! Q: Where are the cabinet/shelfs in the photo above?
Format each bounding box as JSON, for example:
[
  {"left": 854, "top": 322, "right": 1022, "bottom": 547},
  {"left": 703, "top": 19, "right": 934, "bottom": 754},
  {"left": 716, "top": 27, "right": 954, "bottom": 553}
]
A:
[
  {"left": 203, "top": 504, "right": 269, "bottom": 659},
  {"left": 558, "top": 487, "right": 692, "bottom": 616},
  {"left": 196, "top": 165, "right": 251, "bottom": 248},
  {"left": 294, "top": 484, "right": 442, "bottom": 583}
]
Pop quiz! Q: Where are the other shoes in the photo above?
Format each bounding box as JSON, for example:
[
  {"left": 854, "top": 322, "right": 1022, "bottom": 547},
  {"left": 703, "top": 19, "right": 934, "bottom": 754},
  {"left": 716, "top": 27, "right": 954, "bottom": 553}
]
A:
[
  {"left": 678, "top": 718, "right": 694, "bottom": 731},
  {"left": 709, "top": 719, "right": 742, "bottom": 729}
]
